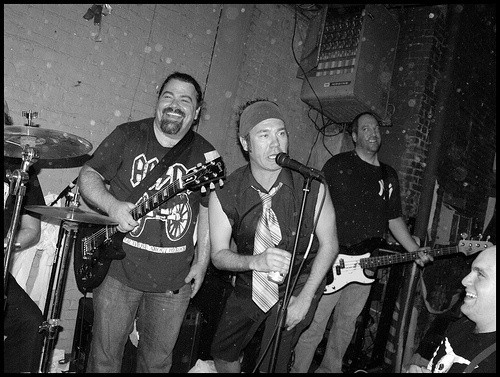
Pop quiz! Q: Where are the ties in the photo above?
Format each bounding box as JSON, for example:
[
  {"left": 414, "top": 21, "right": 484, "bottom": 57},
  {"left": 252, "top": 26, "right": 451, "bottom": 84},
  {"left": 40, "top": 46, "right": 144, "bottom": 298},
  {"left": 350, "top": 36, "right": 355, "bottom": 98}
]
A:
[{"left": 250, "top": 182, "right": 280, "bottom": 313}]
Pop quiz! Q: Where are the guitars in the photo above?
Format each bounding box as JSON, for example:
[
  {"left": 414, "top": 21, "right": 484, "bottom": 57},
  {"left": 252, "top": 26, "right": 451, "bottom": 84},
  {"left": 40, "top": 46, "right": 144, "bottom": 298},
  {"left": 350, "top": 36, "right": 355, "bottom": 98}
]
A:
[
  {"left": 324, "top": 232, "right": 494, "bottom": 295},
  {"left": 74, "top": 162, "right": 226, "bottom": 290}
]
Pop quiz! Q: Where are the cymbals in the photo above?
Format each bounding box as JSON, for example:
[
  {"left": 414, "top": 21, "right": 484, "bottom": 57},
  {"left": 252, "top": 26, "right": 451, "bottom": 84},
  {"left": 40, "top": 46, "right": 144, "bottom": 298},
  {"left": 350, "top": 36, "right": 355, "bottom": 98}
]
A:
[
  {"left": 4, "top": 124, "right": 93, "bottom": 159},
  {"left": 23, "top": 205, "right": 119, "bottom": 225}
]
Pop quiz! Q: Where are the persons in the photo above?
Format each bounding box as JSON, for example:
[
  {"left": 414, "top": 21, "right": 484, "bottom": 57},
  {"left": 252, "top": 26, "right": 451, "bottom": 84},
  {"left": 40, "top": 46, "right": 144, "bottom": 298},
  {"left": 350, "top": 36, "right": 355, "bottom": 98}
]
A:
[
  {"left": 77, "top": 72, "right": 225, "bottom": 373},
  {"left": 208, "top": 99, "right": 340, "bottom": 373},
  {"left": 285, "top": 114, "right": 433, "bottom": 372},
  {"left": 4, "top": 115, "right": 45, "bottom": 373},
  {"left": 404, "top": 245, "right": 497, "bottom": 373}
]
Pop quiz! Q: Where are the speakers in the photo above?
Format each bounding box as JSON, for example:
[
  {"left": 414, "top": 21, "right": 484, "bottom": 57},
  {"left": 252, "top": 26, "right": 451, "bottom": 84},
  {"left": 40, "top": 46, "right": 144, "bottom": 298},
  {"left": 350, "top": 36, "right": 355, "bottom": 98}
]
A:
[{"left": 69, "top": 297, "right": 204, "bottom": 373}]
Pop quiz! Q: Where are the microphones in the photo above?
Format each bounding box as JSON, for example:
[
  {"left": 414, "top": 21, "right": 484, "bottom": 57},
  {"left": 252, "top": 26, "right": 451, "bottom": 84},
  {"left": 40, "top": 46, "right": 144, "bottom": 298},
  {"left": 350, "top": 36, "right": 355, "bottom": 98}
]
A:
[
  {"left": 275, "top": 152, "right": 327, "bottom": 183},
  {"left": 58, "top": 176, "right": 79, "bottom": 200}
]
